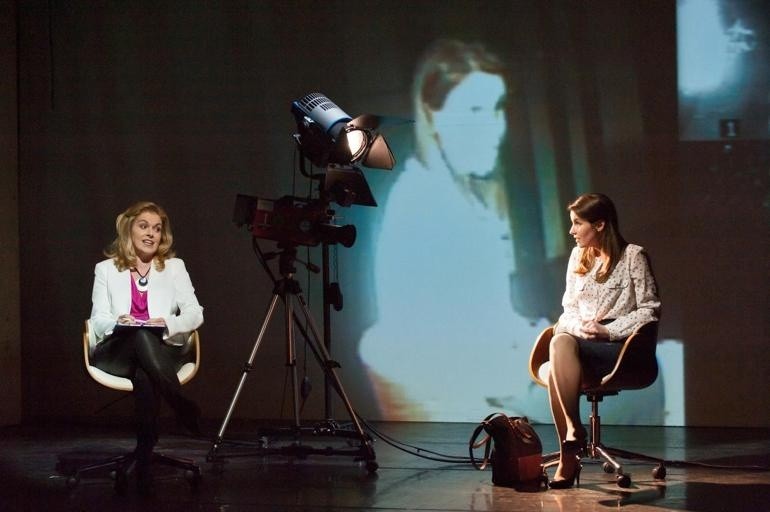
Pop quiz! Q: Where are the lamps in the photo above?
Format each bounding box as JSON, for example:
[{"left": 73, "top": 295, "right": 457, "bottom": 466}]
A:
[{"left": 295, "top": 90, "right": 370, "bottom": 164}]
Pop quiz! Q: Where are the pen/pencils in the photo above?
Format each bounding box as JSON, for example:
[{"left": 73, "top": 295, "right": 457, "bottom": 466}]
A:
[{"left": 140, "top": 322, "right": 144, "bottom": 327}]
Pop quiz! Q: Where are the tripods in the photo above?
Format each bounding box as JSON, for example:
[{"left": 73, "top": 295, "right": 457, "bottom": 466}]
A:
[{"left": 207, "top": 276, "right": 379, "bottom": 473}]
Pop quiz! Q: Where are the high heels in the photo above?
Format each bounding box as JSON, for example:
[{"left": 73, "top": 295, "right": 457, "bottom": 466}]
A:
[
  {"left": 562, "top": 428, "right": 589, "bottom": 458},
  {"left": 549, "top": 459, "right": 582, "bottom": 488}
]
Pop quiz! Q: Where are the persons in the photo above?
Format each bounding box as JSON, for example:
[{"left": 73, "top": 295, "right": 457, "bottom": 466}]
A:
[
  {"left": 375, "top": 39, "right": 664, "bottom": 426},
  {"left": 88, "top": 201, "right": 205, "bottom": 492},
  {"left": 547, "top": 192, "right": 660, "bottom": 489}
]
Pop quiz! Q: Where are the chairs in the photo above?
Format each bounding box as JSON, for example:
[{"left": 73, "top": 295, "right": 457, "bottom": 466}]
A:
[
  {"left": 56, "top": 328, "right": 202, "bottom": 492},
  {"left": 530, "top": 320, "right": 667, "bottom": 488}
]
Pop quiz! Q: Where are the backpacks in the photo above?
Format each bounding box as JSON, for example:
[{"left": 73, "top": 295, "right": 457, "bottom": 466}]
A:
[{"left": 469, "top": 412, "right": 548, "bottom": 492}]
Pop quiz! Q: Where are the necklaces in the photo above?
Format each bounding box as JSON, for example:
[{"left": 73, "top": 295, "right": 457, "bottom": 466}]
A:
[{"left": 134, "top": 266, "right": 151, "bottom": 285}]
